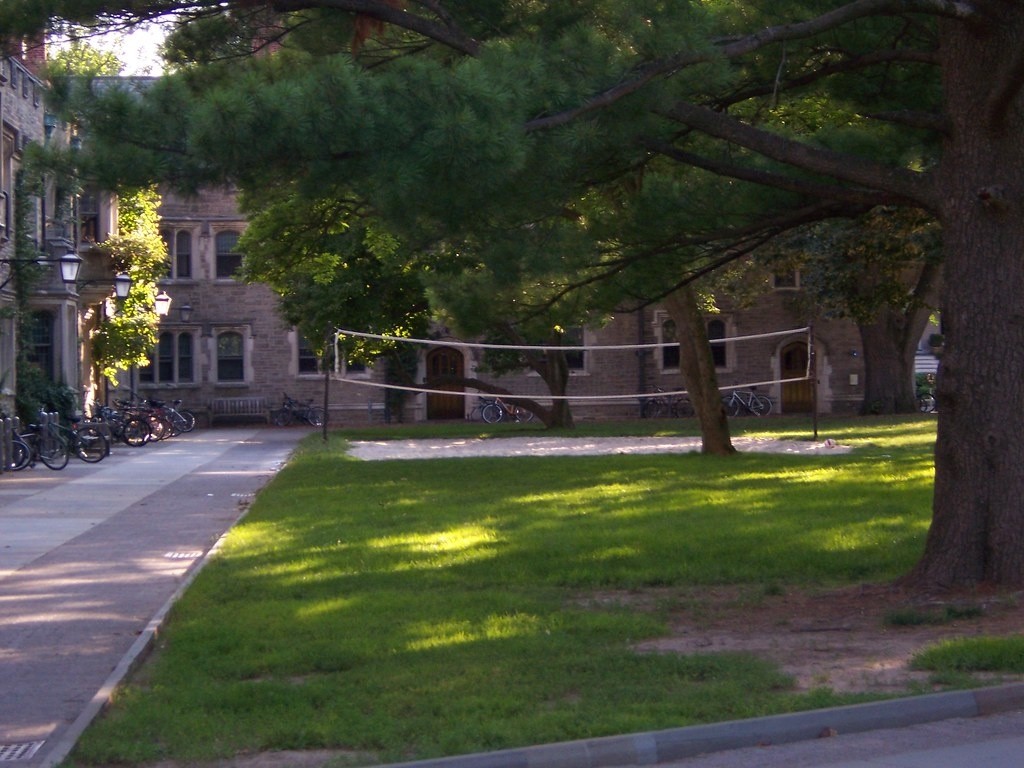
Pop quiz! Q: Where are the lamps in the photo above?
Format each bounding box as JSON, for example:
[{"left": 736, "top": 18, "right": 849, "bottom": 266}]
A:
[{"left": 0, "top": 249, "right": 195, "bottom": 322}]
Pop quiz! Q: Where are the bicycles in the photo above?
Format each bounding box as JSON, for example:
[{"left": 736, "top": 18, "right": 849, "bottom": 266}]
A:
[
  {"left": 916, "top": 384, "right": 936, "bottom": 413},
  {"left": 721, "top": 381, "right": 773, "bottom": 418},
  {"left": 643, "top": 385, "right": 693, "bottom": 419},
  {"left": 3, "top": 424, "right": 69, "bottom": 471},
  {"left": 481, "top": 398, "right": 534, "bottom": 424},
  {"left": 49, "top": 417, "right": 109, "bottom": 463},
  {"left": 275, "top": 392, "right": 329, "bottom": 427},
  {"left": 84, "top": 391, "right": 196, "bottom": 447}
]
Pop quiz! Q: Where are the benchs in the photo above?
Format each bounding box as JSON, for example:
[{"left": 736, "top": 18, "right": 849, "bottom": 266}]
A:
[{"left": 208, "top": 396, "right": 271, "bottom": 426}]
